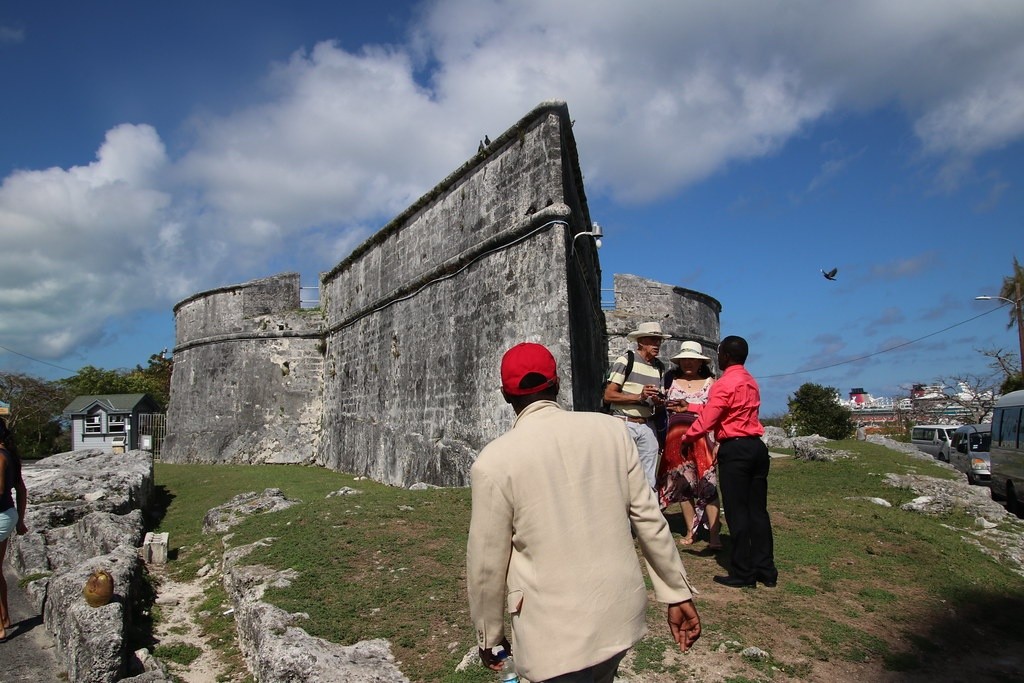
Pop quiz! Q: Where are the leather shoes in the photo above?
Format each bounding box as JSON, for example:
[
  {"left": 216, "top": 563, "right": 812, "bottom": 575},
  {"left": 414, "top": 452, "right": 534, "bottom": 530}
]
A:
[
  {"left": 758, "top": 575, "right": 778, "bottom": 587},
  {"left": 713, "top": 573, "right": 757, "bottom": 587}
]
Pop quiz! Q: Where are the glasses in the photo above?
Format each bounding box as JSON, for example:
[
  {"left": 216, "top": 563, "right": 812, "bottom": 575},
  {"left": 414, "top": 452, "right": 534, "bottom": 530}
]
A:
[
  {"left": 556, "top": 377, "right": 560, "bottom": 383},
  {"left": 652, "top": 337, "right": 666, "bottom": 343}
]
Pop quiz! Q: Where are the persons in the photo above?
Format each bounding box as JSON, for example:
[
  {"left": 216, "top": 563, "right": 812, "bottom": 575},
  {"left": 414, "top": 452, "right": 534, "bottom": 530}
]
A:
[
  {"left": 0, "top": 416, "right": 29, "bottom": 644},
  {"left": 604, "top": 322, "right": 668, "bottom": 513},
  {"left": 466, "top": 342, "right": 702, "bottom": 683},
  {"left": 667, "top": 336, "right": 778, "bottom": 589},
  {"left": 659, "top": 340, "right": 723, "bottom": 548}
]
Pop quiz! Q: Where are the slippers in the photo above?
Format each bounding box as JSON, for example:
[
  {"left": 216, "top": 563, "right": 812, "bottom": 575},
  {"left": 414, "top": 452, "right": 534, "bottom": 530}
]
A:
[
  {"left": 679, "top": 535, "right": 695, "bottom": 545},
  {"left": 709, "top": 540, "right": 722, "bottom": 549}
]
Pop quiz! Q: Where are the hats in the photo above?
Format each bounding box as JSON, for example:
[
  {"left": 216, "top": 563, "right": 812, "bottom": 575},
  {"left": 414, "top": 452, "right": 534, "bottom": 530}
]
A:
[
  {"left": 627, "top": 322, "right": 673, "bottom": 342},
  {"left": 670, "top": 340, "right": 711, "bottom": 364},
  {"left": 500, "top": 342, "right": 561, "bottom": 395}
]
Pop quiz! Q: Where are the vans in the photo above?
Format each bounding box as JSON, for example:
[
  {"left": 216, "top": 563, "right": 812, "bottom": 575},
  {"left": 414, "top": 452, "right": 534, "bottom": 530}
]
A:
[
  {"left": 912, "top": 425, "right": 963, "bottom": 461},
  {"left": 950, "top": 424, "right": 990, "bottom": 485},
  {"left": 991, "top": 390, "right": 1023, "bottom": 512}
]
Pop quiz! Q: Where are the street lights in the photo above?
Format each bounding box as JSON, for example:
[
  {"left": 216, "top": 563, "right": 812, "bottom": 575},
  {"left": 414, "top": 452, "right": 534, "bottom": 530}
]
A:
[{"left": 975, "top": 282, "right": 1024, "bottom": 380}]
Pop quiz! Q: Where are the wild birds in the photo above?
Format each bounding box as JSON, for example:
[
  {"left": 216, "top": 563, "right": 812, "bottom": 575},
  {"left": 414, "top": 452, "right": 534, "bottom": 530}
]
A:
[
  {"left": 478, "top": 135, "right": 491, "bottom": 153},
  {"left": 821, "top": 268, "right": 838, "bottom": 281}
]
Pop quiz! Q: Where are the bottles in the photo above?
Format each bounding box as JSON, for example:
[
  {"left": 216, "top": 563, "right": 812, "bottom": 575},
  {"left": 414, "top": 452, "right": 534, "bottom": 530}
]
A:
[{"left": 497, "top": 650, "right": 520, "bottom": 683}]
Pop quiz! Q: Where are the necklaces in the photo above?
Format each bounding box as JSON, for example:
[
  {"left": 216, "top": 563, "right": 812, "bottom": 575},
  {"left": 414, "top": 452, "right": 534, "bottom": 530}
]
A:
[{"left": 688, "top": 384, "right": 691, "bottom": 388}]
